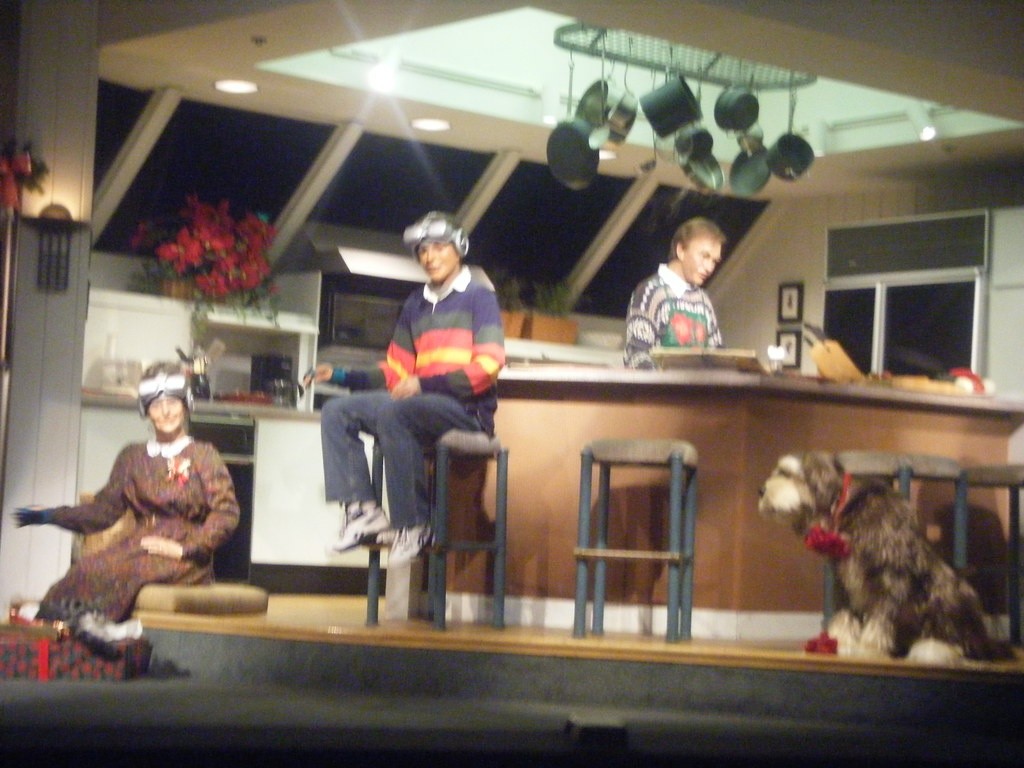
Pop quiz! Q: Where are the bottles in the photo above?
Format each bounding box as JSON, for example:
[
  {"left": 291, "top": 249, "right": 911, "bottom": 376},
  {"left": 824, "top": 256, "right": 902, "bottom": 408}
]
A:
[{"left": 193, "top": 345, "right": 211, "bottom": 400}]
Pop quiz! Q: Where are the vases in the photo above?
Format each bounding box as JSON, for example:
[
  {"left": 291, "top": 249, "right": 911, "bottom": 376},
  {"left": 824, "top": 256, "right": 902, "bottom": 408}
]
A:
[{"left": 154, "top": 275, "right": 209, "bottom": 301}]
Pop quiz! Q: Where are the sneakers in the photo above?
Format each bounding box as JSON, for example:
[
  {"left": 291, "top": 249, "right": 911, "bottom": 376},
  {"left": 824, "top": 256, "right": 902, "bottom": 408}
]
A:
[
  {"left": 387, "top": 523, "right": 431, "bottom": 569},
  {"left": 333, "top": 499, "right": 391, "bottom": 552}
]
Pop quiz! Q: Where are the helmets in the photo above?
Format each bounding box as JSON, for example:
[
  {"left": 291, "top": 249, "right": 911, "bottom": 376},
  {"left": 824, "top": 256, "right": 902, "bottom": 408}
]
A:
[
  {"left": 402, "top": 210, "right": 469, "bottom": 265},
  {"left": 137, "top": 361, "right": 196, "bottom": 421}
]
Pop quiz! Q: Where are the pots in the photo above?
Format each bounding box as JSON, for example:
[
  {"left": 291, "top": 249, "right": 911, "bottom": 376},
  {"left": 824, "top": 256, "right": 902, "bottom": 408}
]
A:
[{"left": 547, "top": 72, "right": 813, "bottom": 197}]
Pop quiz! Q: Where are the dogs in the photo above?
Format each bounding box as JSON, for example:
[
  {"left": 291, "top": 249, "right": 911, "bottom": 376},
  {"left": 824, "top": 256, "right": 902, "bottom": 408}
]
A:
[{"left": 757, "top": 450, "right": 1016, "bottom": 665}]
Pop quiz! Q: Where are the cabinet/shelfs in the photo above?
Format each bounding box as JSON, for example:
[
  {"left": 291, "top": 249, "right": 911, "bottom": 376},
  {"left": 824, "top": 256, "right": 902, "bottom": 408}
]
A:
[{"left": 74, "top": 251, "right": 439, "bottom": 569}]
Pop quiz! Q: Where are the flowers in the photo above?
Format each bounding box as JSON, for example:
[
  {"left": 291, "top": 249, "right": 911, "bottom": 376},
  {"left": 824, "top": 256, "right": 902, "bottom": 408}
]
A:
[{"left": 121, "top": 191, "right": 274, "bottom": 297}]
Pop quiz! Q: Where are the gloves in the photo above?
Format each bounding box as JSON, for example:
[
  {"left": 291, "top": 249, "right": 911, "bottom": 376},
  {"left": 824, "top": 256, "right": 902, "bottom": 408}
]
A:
[{"left": 13, "top": 507, "right": 49, "bottom": 529}]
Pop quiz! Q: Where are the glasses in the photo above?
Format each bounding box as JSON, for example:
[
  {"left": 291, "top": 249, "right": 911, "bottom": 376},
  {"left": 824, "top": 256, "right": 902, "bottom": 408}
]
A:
[
  {"left": 404, "top": 219, "right": 447, "bottom": 245},
  {"left": 138, "top": 373, "right": 186, "bottom": 396}
]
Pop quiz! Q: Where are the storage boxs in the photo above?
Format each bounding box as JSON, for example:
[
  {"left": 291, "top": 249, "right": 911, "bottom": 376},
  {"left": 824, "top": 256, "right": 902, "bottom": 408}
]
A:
[{"left": 319, "top": 291, "right": 405, "bottom": 349}]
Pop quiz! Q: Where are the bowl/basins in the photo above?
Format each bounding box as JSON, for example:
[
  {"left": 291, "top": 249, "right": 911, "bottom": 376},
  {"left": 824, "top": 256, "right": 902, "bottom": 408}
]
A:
[{"left": 580, "top": 330, "right": 623, "bottom": 351}]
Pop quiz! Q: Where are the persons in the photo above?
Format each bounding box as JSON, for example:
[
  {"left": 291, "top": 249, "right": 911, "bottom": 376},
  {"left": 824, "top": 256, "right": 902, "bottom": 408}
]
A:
[
  {"left": 304, "top": 211, "right": 505, "bottom": 564},
  {"left": 12, "top": 360, "right": 240, "bottom": 625},
  {"left": 622, "top": 217, "right": 726, "bottom": 372}
]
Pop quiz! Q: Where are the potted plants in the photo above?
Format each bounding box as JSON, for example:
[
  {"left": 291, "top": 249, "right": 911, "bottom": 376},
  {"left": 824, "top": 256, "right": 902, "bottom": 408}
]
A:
[
  {"left": 484, "top": 267, "right": 525, "bottom": 338},
  {"left": 522, "top": 280, "right": 581, "bottom": 346}
]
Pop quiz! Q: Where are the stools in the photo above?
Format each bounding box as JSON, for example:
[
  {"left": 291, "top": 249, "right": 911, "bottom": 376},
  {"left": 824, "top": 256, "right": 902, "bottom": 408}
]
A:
[
  {"left": 816, "top": 454, "right": 962, "bottom": 655},
  {"left": 950, "top": 461, "right": 1024, "bottom": 659},
  {"left": 363, "top": 427, "right": 509, "bottom": 634},
  {"left": 571, "top": 437, "right": 701, "bottom": 643}
]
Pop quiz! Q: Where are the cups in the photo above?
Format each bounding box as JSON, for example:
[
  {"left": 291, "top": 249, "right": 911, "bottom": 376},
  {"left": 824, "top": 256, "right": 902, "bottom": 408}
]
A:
[{"left": 267, "top": 377, "right": 292, "bottom": 406}]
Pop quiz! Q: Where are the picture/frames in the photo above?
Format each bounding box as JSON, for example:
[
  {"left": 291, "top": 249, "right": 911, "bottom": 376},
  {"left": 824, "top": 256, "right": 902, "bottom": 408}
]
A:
[
  {"left": 778, "top": 281, "right": 802, "bottom": 323},
  {"left": 776, "top": 330, "right": 801, "bottom": 368}
]
